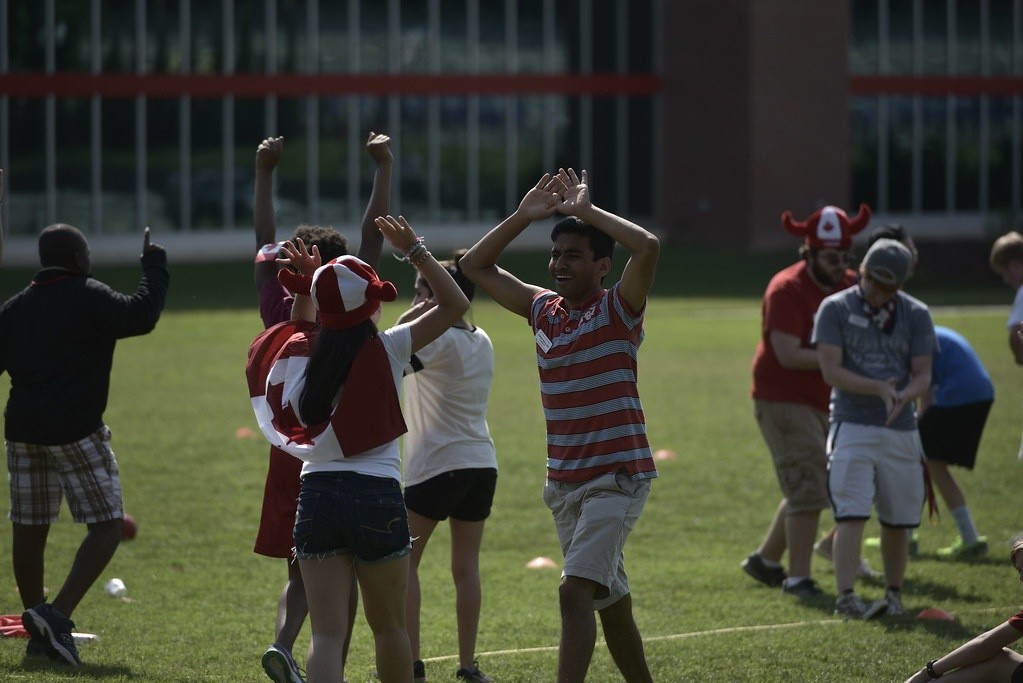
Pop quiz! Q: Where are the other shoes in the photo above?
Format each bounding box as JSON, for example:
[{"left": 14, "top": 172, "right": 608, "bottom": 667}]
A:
[
  {"left": 834, "top": 591, "right": 867, "bottom": 619},
  {"left": 884, "top": 590, "right": 904, "bottom": 618},
  {"left": 814, "top": 543, "right": 882, "bottom": 579}
]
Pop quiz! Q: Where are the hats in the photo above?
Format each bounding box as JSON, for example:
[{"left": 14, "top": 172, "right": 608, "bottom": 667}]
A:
[
  {"left": 782, "top": 204, "right": 870, "bottom": 249},
  {"left": 280, "top": 255, "right": 398, "bottom": 330},
  {"left": 863, "top": 239, "right": 913, "bottom": 289}
]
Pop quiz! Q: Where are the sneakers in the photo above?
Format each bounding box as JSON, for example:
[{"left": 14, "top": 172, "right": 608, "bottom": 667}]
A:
[
  {"left": 937, "top": 535, "right": 987, "bottom": 559},
  {"left": 262, "top": 643, "right": 305, "bottom": 683},
  {"left": 866, "top": 534, "right": 919, "bottom": 553},
  {"left": 742, "top": 554, "right": 785, "bottom": 588},
  {"left": 782, "top": 580, "right": 827, "bottom": 605},
  {"left": 374, "top": 660, "right": 426, "bottom": 683},
  {"left": 22, "top": 603, "right": 78, "bottom": 666},
  {"left": 457, "top": 655, "right": 496, "bottom": 683}
]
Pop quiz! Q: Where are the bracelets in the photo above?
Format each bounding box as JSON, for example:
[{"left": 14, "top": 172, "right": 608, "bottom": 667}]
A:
[
  {"left": 392, "top": 236, "right": 431, "bottom": 269},
  {"left": 926, "top": 659, "right": 943, "bottom": 680}
]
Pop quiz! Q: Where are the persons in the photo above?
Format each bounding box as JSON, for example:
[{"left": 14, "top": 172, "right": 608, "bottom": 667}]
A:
[
  {"left": 0, "top": 221, "right": 172, "bottom": 666},
  {"left": 903, "top": 539, "right": 1023, "bottom": 683},
  {"left": 245, "top": 130, "right": 395, "bottom": 683},
  {"left": 395, "top": 248, "right": 499, "bottom": 683},
  {"left": 989, "top": 232, "right": 1022, "bottom": 367},
  {"left": 457, "top": 168, "right": 661, "bottom": 683},
  {"left": 739, "top": 202, "right": 994, "bottom": 619},
  {"left": 809, "top": 238, "right": 938, "bottom": 618},
  {"left": 246, "top": 213, "right": 472, "bottom": 683}
]
